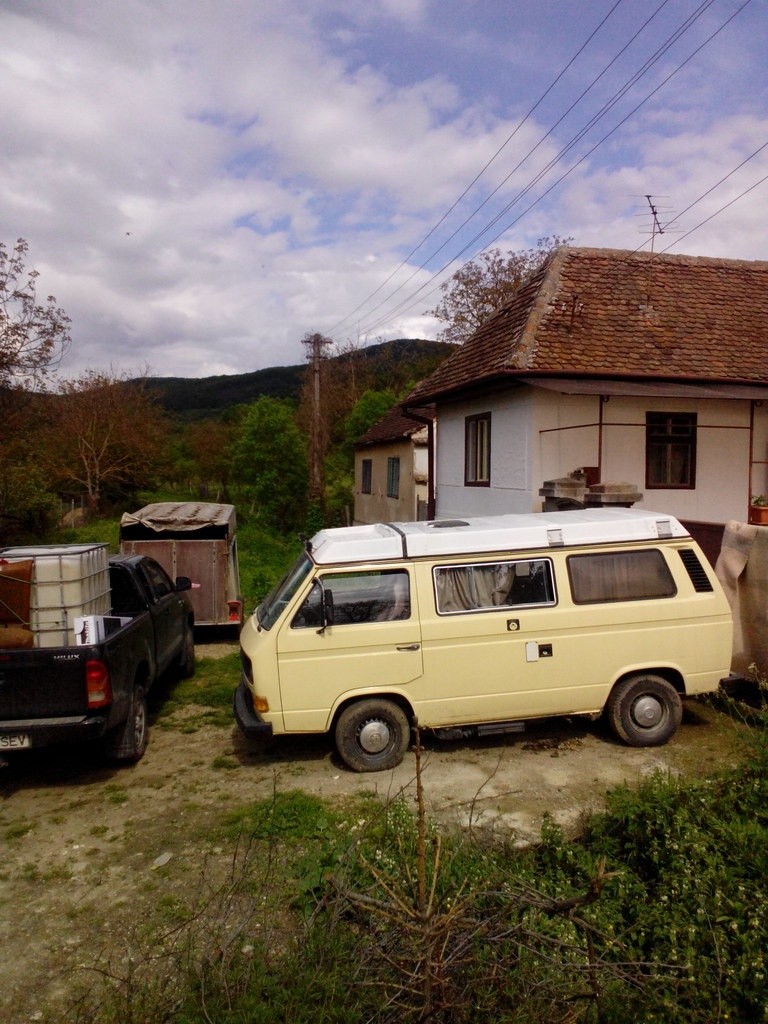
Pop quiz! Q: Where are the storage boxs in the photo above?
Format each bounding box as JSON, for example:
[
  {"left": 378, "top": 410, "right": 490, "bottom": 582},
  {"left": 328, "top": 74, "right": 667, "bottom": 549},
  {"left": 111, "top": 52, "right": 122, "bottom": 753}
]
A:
[{"left": 73, "top": 615, "right": 133, "bottom": 646}]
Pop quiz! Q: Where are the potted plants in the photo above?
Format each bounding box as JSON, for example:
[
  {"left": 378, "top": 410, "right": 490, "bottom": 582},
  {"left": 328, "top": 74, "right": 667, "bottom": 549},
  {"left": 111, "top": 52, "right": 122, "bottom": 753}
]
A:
[{"left": 749, "top": 493, "right": 768, "bottom": 526}]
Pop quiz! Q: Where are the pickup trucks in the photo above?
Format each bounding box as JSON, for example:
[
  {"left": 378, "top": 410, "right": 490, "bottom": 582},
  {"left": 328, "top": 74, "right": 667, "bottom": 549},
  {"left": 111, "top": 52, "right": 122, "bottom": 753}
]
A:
[{"left": 1, "top": 555, "right": 196, "bottom": 764}]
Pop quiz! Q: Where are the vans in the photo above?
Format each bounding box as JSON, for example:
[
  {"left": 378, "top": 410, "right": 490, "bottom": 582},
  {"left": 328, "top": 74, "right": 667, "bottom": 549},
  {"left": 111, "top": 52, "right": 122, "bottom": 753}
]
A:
[{"left": 232, "top": 507, "right": 734, "bottom": 774}]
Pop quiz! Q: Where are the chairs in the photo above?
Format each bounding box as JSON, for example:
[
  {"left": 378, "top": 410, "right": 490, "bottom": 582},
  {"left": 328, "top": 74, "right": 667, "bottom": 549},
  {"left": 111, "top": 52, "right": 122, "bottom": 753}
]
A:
[{"left": 369, "top": 574, "right": 407, "bottom": 621}]
